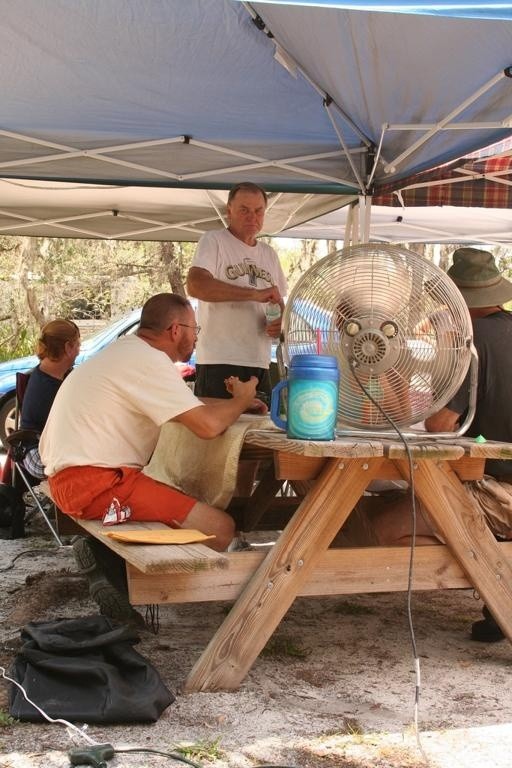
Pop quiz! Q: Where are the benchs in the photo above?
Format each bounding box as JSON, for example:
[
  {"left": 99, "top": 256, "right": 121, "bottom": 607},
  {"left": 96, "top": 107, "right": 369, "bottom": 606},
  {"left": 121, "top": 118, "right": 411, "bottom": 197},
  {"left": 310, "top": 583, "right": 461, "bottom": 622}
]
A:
[{"left": 39, "top": 479, "right": 268, "bottom": 606}]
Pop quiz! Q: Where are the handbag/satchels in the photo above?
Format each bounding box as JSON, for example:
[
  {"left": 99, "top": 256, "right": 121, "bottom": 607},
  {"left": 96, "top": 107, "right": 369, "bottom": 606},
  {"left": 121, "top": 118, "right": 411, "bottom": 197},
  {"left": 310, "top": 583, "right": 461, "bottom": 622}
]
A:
[{"left": 11, "top": 615, "right": 175, "bottom": 728}]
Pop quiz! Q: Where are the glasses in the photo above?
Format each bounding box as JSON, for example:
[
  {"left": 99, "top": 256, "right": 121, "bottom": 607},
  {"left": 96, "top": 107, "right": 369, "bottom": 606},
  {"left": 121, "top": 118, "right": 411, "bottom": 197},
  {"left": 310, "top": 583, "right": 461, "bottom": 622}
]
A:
[{"left": 166, "top": 321, "right": 201, "bottom": 337}]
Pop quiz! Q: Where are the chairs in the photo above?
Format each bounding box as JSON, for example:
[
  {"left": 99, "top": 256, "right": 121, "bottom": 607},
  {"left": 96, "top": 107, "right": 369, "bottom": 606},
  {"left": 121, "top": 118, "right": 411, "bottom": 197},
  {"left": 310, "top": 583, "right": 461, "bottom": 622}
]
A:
[{"left": 11, "top": 372, "right": 64, "bottom": 546}]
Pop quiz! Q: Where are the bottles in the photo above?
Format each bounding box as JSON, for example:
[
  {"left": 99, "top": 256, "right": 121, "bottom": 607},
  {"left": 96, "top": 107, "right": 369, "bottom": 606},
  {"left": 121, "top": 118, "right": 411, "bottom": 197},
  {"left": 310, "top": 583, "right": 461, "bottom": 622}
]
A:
[
  {"left": 363, "top": 373, "right": 383, "bottom": 424},
  {"left": 266, "top": 300, "right": 282, "bottom": 337}
]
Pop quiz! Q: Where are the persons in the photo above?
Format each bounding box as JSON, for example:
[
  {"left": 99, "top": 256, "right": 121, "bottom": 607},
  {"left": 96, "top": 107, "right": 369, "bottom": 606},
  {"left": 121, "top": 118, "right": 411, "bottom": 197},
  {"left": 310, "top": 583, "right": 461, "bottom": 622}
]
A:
[
  {"left": 325, "top": 285, "right": 419, "bottom": 432},
  {"left": 372, "top": 246, "right": 512, "bottom": 644},
  {"left": 16, "top": 318, "right": 81, "bottom": 480},
  {"left": 187, "top": 181, "right": 289, "bottom": 551},
  {"left": 411, "top": 273, "right": 457, "bottom": 361},
  {"left": 37, "top": 292, "right": 260, "bottom": 628}
]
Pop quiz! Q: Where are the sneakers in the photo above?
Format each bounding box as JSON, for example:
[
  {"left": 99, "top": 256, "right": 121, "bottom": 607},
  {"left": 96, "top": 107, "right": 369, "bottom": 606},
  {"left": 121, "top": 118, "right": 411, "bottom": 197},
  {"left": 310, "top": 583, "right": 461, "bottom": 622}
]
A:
[
  {"left": 90, "top": 579, "right": 145, "bottom": 630},
  {"left": 469, "top": 602, "right": 507, "bottom": 643},
  {"left": 70, "top": 532, "right": 116, "bottom": 594}
]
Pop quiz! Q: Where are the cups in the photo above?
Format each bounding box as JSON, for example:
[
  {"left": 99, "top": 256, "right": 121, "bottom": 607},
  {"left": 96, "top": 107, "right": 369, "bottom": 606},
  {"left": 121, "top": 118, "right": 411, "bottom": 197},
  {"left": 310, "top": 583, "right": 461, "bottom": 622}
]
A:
[{"left": 270, "top": 354, "right": 340, "bottom": 440}]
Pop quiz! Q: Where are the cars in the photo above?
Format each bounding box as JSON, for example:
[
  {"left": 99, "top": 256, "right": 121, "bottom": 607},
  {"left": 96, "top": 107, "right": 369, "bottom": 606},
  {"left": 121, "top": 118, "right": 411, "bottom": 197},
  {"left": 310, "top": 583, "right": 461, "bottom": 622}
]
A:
[{"left": 0, "top": 296, "right": 437, "bottom": 453}]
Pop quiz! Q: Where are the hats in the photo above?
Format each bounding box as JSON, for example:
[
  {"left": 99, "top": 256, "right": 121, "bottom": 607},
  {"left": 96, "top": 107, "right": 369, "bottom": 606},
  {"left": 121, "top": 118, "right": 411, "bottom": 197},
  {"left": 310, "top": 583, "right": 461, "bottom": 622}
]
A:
[{"left": 423, "top": 247, "right": 512, "bottom": 308}]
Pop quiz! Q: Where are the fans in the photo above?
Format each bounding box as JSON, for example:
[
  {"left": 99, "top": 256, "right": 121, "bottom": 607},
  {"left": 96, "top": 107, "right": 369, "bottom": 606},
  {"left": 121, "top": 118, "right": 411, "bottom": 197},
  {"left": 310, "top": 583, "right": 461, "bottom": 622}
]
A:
[{"left": 276, "top": 244, "right": 478, "bottom": 438}]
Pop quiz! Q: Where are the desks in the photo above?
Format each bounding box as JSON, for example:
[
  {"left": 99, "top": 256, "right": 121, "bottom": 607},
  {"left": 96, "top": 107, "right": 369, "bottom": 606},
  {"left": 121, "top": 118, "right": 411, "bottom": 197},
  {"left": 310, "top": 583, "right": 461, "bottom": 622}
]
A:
[{"left": 179, "top": 413, "right": 512, "bottom": 693}]
